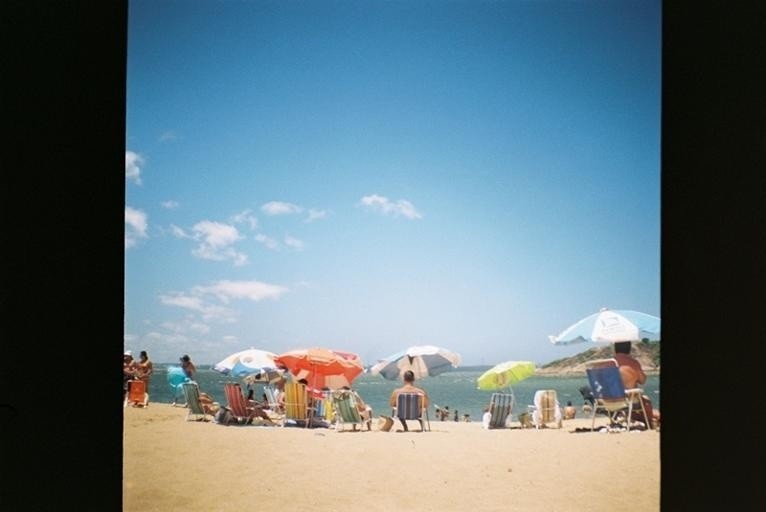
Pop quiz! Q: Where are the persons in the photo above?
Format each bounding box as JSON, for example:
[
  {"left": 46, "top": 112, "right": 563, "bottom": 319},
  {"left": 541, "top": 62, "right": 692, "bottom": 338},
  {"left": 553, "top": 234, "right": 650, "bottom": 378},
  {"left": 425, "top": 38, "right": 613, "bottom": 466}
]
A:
[
  {"left": 582, "top": 400, "right": 593, "bottom": 416},
  {"left": 454, "top": 410, "right": 459, "bottom": 421},
  {"left": 123, "top": 351, "right": 135, "bottom": 404},
  {"left": 195, "top": 376, "right": 373, "bottom": 433},
  {"left": 611, "top": 340, "right": 656, "bottom": 430},
  {"left": 483, "top": 390, "right": 511, "bottom": 418},
  {"left": 441, "top": 405, "right": 449, "bottom": 420},
  {"left": 463, "top": 412, "right": 470, "bottom": 422},
  {"left": 133, "top": 351, "right": 153, "bottom": 408},
  {"left": 171, "top": 355, "right": 197, "bottom": 408},
  {"left": 435, "top": 408, "right": 441, "bottom": 419},
  {"left": 564, "top": 400, "right": 576, "bottom": 418},
  {"left": 390, "top": 370, "right": 429, "bottom": 432}
]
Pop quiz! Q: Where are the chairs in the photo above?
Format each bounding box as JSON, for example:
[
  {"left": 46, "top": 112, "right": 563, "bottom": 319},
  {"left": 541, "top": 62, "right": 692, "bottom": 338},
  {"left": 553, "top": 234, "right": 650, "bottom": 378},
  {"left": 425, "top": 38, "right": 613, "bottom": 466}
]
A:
[
  {"left": 182, "top": 383, "right": 216, "bottom": 422},
  {"left": 332, "top": 389, "right": 372, "bottom": 432},
  {"left": 483, "top": 393, "right": 514, "bottom": 430},
  {"left": 392, "top": 392, "right": 428, "bottom": 433},
  {"left": 263, "top": 385, "right": 283, "bottom": 414},
  {"left": 224, "top": 381, "right": 272, "bottom": 426},
  {"left": 283, "top": 383, "right": 316, "bottom": 429},
  {"left": 528, "top": 390, "right": 562, "bottom": 430},
  {"left": 584, "top": 358, "right": 651, "bottom": 433},
  {"left": 579, "top": 386, "right": 631, "bottom": 428},
  {"left": 123, "top": 380, "right": 149, "bottom": 409}
]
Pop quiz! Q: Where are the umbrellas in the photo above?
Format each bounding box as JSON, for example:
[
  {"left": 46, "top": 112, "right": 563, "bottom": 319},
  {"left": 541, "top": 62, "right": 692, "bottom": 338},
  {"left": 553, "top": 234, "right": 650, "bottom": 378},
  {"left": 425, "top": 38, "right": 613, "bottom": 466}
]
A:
[
  {"left": 549, "top": 307, "right": 661, "bottom": 356},
  {"left": 242, "top": 370, "right": 287, "bottom": 386},
  {"left": 275, "top": 346, "right": 364, "bottom": 427},
  {"left": 476, "top": 360, "right": 537, "bottom": 390},
  {"left": 365, "top": 344, "right": 462, "bottom": 425},
  {"left": 207, "top": 345, "right": 283, "bottom": 390}
]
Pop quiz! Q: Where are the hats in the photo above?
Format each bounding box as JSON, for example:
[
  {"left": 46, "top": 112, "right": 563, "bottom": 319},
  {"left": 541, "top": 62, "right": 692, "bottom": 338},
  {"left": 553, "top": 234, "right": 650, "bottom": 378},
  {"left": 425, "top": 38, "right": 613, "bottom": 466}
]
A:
[
  {"left": 123, "top": 351, "right": 132, "bottom": 356},
  {"left": 404, "top": 370, "right": 414, "bottom": 380},
  {"left": 180, "top": 354, "right": 190, "bottom": 362}
]
